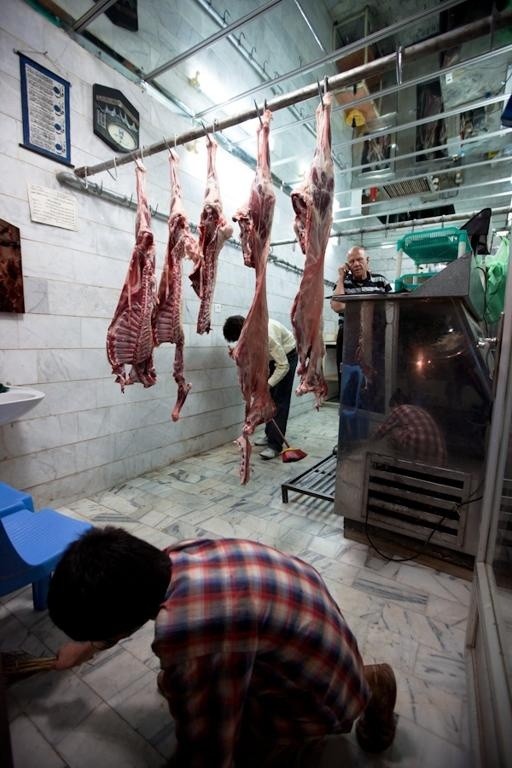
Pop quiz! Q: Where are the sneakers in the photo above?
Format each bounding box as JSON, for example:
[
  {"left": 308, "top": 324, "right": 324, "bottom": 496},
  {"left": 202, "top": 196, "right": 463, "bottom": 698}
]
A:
[
  {"left": 258, "top": 448, "right": 280, "bottom": 458},
  {"left": 355, "top": 663, "right": 397, "bottom": 754},
  {"left": 254, "top": 437, "right": 268, "bottom": 445}
]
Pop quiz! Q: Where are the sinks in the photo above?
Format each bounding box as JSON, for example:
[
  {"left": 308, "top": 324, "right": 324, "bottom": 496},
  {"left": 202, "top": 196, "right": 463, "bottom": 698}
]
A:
[{"left": 0, "top": 385, "right": 46, "bottom": 426}]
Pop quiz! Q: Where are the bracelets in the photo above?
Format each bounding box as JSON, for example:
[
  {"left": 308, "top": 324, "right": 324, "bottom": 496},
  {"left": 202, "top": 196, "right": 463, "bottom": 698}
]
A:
[{"left": 89, "top": 642, "right": 107, "bottom": 651}]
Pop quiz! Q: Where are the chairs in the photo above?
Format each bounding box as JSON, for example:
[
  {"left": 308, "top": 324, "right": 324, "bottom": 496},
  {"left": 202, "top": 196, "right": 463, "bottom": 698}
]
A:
[
  {"left": 0, "top": 507, "right": 94, "bottom": 611},
  {"left": 0, "top": 481, "right": 34, "bottom": 517}
]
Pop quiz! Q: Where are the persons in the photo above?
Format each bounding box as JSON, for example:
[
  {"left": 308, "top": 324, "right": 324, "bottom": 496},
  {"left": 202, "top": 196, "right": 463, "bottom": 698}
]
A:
[
  {"left": 49, "top": 525, "right": 396, "bottom": 768},
  {"left": 330, "top": 245, "right": 392, "bottom": 399},
  {"left": 223, "top": 315, "right": 298, "bottom": 459},
  {"left": 369, "top": 390, "right": 448, "bottom": 465}
]
